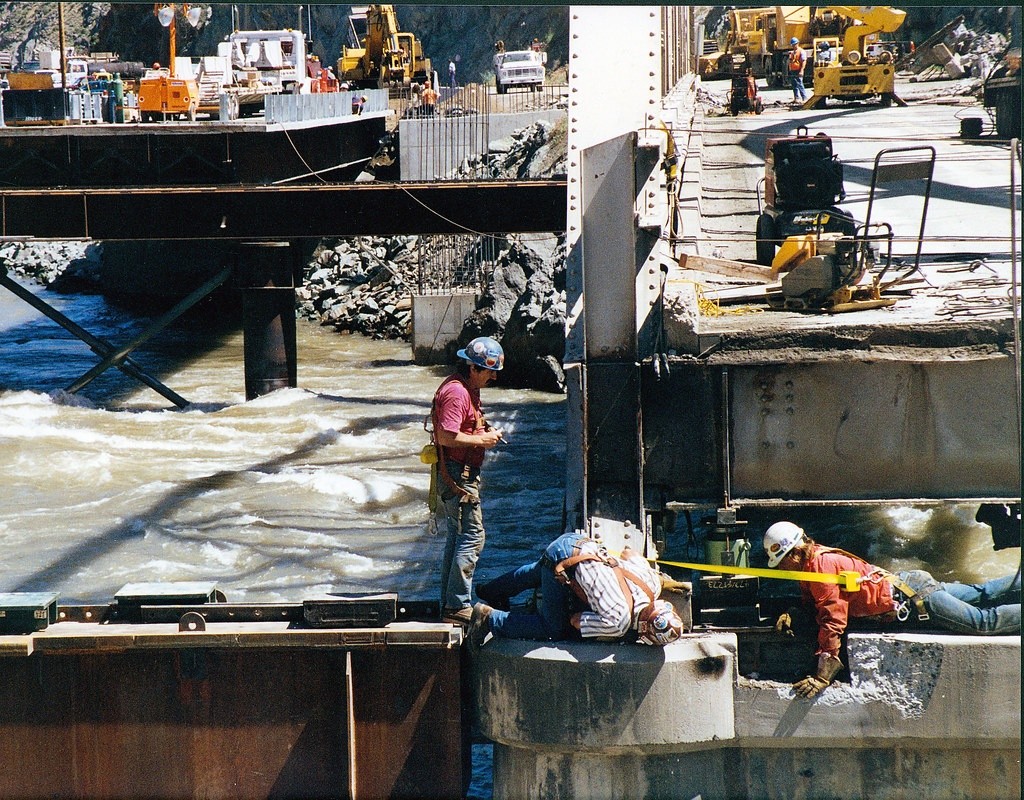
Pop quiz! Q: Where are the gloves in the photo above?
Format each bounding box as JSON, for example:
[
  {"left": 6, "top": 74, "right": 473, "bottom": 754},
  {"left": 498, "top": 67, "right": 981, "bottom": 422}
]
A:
[
  {"left": 793, "top": 653, "right": 844, "bottom": 699},
  {"left": 659, "top": 576, "right": 691, "bottom": 594},
  {"left": 775, "top": 606, "right": 800, "bottom": 636}
]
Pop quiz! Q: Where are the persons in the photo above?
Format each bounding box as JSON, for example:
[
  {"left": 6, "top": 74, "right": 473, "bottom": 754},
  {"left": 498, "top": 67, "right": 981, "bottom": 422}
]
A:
[
  {"left": 421, "top": 337, "right": 508, "bottom": 626},
  {"left": 419, "top": 80, "right": 441, "bottom": 117},
  {"left": 448, "top": 58, "right": 457, "bottom": 88},
  {"left": 817, "top": 40, "right": 836, "bottom": 61},
  {"left": 460, "top": 533, "right": 692, "bottom": 657},
  {"left": 339, "top": 81, "right": 367, "bottom": 116},
  {"left": 763, "top": 520, "right": 1022, "bottom": 698},
  {"left": 787, "top": 37, "right": 808, "bottom": 101}
]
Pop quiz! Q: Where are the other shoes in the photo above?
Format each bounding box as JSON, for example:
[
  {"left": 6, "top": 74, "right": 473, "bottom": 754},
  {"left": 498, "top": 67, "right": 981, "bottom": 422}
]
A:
[
  {"left": 467, "top": 602, "right": 493, "bottom": 657},
  {"left": 443, "top": 606, "right": 474, "bottom": 625}
]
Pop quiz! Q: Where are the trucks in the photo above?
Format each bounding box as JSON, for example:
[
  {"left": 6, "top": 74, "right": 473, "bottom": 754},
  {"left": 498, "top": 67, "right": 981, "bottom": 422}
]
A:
[{"left": 493, "top": 49, "right": 548, "bottom": 94}]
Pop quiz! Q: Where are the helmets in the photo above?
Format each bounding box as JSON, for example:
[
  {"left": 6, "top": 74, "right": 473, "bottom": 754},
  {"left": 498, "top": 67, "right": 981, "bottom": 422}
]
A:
[
  {"left": 637, "top": 599, "right": 683, "bottom": 647},
  {"left": 791, "top": 37, "right": 800, "bottom": 45},
  {"left": 425, "top": 81, "right": 430, "bottom": 87},
  {"left": 457, "top": 337, "right": 504, "bottom": 370},
  {"left": 153, "top": 63, "right": 160, "bottom": 69},
  {"left": 819, "top": 42, "right": 830, "bottom": 49},
  {"left": 340, "top": 83, "right": 349, "bottom": 89},
  {"left": 764, "top": 521, "right": 804, "bottom": 567}
]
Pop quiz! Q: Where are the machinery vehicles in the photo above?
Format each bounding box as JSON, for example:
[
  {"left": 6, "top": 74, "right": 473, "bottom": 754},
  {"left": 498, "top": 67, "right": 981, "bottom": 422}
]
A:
[
  {"left": 0, "top": 1, "right": 338, "bottom": 125},
  {"left": 698, "top": 5, "right": 907, "bottom": 111},
  {"left": 335, "top": 4, "right": 430, "bottom": 101}
]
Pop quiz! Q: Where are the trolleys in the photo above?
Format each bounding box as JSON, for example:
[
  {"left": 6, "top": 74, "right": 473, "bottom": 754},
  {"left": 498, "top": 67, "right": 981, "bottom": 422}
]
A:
[{"left": 760, "top": 144, "right": 938, "bottom": 315}]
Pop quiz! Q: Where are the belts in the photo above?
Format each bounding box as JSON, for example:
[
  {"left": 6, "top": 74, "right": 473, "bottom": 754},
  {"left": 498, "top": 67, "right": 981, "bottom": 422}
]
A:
[
  {"left": 572, "top": 537, "right": 594, "bottom": 556},
  {"left": 892, "top": 586, "right": 900, "bottom": 602}
]
